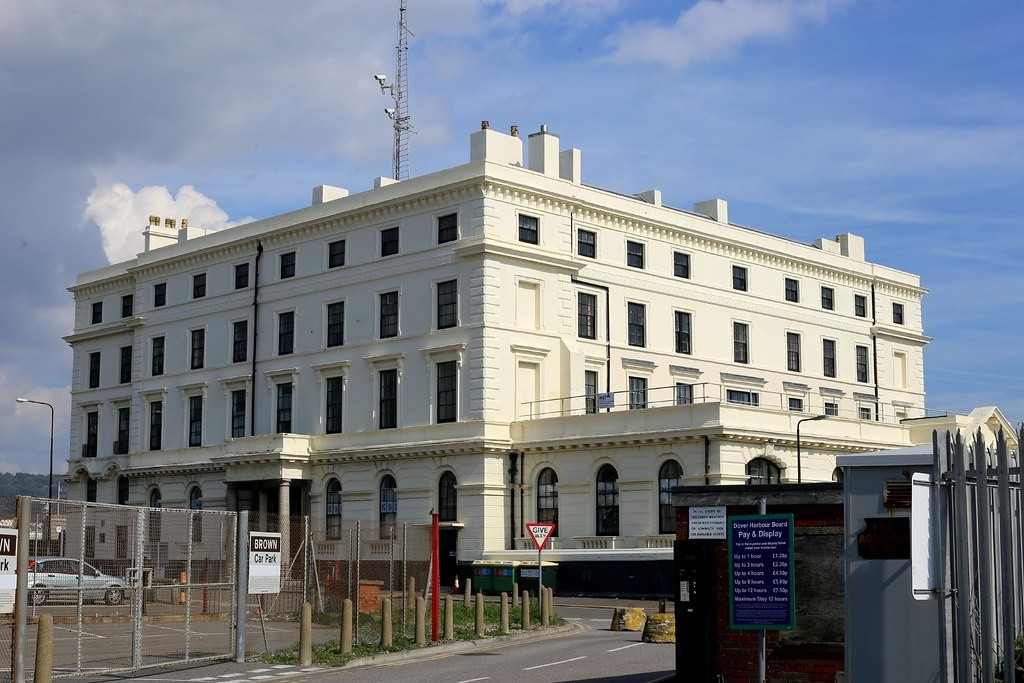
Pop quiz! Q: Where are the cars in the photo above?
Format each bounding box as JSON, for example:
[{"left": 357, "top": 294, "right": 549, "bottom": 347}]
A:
[{"left": 26, "top": 556, "right": 131, "bottom": 604}]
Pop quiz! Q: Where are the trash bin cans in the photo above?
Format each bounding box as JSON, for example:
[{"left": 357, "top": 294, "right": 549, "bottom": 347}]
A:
[
  {"left": 470, "top": 559, "right": 493, "bottom": 594},
  {"left": 518, "top": 561, "right": 560, "bottom": 598},
  {"left": 489, "top": 560, "right": 521, "bottom": 597}
]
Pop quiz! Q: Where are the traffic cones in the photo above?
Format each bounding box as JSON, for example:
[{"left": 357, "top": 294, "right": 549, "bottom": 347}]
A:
[{"left": 451, "top": 574, "right": 462, "bottom": 595}]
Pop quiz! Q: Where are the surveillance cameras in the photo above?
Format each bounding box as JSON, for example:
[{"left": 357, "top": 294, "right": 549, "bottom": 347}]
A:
[
  {"left": 374, "top": 75, "right": 386, "bottom": 80},
  {"left": 384, "top": 108, "right": 396, "bottom": 114}
]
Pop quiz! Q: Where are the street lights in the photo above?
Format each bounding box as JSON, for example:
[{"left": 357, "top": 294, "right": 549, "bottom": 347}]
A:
[
  {"left": 797, "top": 415, "right": 828, "bottom": 482},
  {"left": 15, "top": 398, "right": 53, "bottom": 556}
]
